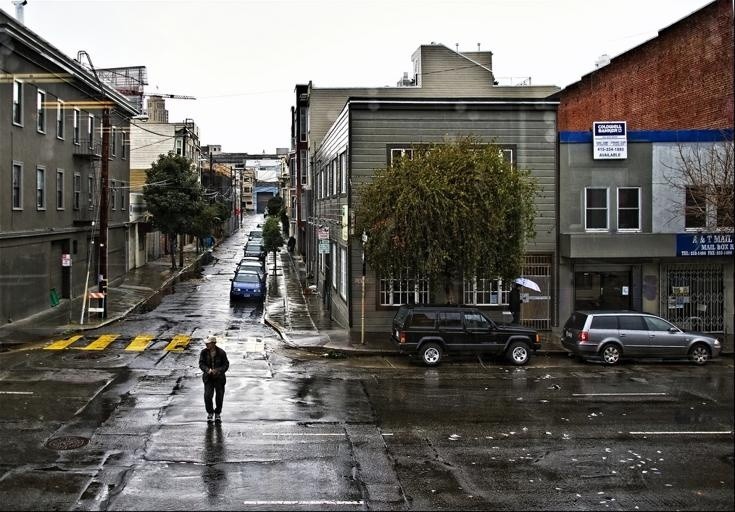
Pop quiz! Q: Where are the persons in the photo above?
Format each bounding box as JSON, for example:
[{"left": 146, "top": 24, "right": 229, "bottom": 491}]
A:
[
  {"left": 509, "top": 285, "right": 523, "bottom": 325},
  {"left": 198, "top": 336, "right": 229, "bottom": 422}
]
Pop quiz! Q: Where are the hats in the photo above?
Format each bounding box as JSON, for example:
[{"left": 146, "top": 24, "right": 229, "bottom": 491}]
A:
[{"left": 203, "top": 335, "right": 216, "bottom": 344}]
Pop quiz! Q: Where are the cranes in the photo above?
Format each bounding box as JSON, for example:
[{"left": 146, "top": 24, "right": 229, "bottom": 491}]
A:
[{"left": 108, "top": 85, "right": 201, "bottom": 101}]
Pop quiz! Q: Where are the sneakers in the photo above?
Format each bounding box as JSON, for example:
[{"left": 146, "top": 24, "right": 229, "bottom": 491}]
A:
[
  {"left": 207, "top": 414, "right": 213, "bottom": 422},
  {"left": 216, "top": 414, "right": 221, "bottom": 422}
]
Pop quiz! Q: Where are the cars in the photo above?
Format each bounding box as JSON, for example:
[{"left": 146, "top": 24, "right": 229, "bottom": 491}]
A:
[
  {"left": 263, "top": 206, "right": 276, "bottom": 218},
  {"left": 230, "top": 221, "right": 274, "bottom": 303}
]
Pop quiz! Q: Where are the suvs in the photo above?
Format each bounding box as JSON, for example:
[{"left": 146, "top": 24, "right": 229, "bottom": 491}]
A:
[
  {"left": 559, "top": 309, "right": 722, "bottom": 364},
  {"left": 390, "top": 303, "right": 542, "bottom": 365}
]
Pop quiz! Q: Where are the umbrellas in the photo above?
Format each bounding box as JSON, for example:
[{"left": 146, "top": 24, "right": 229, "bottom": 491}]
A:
[{"left": 511, "top": 277, "right": 542, "bottom": 292}]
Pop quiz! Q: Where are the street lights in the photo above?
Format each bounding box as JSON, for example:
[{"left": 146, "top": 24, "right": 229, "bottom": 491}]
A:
[
  {"left": 97, "top": 106, "right": 151, "bottom": 312},
  {"left": 229, "top": 166, "right": 252, "bottom": 222}
]
